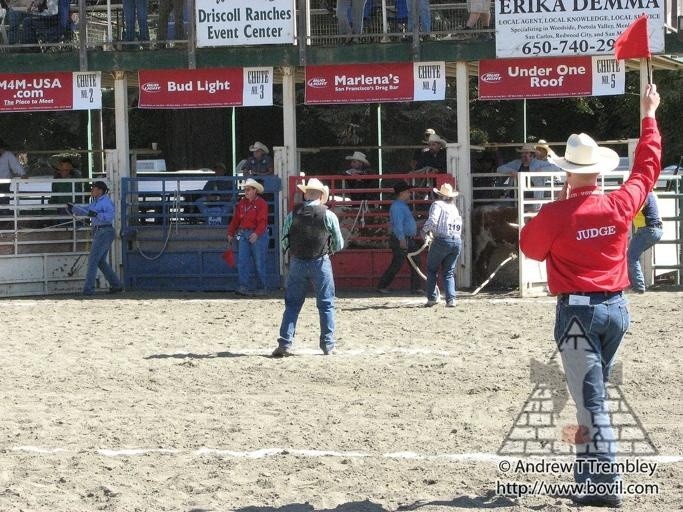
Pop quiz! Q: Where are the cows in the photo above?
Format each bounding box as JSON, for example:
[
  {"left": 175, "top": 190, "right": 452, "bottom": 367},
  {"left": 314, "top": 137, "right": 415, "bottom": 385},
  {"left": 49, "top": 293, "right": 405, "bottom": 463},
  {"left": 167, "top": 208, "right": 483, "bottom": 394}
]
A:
[
  {"left": 328, "top": 206, "right": 428, "bottom": 249},
  {"left": 471, "top": 203, "right": 541, "bottom": 287}
]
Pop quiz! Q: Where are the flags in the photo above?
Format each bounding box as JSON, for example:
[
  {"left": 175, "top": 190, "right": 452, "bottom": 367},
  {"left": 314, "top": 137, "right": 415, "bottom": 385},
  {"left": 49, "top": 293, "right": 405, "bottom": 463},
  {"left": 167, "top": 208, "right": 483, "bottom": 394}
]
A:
[{"left": 612, "top": 13, "right": 650, "bottom": 61}]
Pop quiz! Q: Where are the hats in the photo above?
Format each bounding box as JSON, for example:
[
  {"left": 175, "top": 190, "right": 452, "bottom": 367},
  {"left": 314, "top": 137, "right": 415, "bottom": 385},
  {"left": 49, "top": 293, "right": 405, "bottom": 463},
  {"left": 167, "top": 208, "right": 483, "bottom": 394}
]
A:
[
  {"left": 238, "top": 178, "right": 263, "bottom": 193},
  {"left": 248, "top": 142, "right": 269, "bottom": 154},
  {"left": 394, "top": 181, "right": 413, "bottom": 191},
  {"left": 88, "top": 181, "right": 106, "bottom": 189},
  {"left": 297, "top": 178, "right": 329, "bottom": 204},
  {"left": 516, "top": 140, "right": 551, "bottom": 152},
  {"left": 213, "top": 163, "right": 225, "bottom": 169},
  {"left": 432, "top": 183, "right": 458, "bottom": 197},
  {"left": 422, "top": 129, "right": 446, "bottom": 149},
  {"left": 553, "top": 133, "right": 620, "bottom": 173},
  {"left": 346, "top": 152, "right": 368, "bottom": 164}
]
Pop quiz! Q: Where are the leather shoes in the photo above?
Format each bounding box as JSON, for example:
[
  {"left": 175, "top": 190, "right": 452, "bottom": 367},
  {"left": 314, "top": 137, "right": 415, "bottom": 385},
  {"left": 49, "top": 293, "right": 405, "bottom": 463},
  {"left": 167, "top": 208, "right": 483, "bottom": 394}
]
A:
[
  {"left": 570, "top": 492, "right": 622, "bottom": 508},
  {"left": 324, "top": 347, "right": 336, "bottom": 355},
  {"left": 272, "top": 347, "right": 290, "bottom": 356}
]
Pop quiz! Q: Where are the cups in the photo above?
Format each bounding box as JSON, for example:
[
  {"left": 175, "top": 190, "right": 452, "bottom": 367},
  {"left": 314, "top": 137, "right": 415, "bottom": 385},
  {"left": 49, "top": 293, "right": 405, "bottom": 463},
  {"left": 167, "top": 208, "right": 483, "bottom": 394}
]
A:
[{"left": 151, "top": 143, "right": 157, "bottom": 151}]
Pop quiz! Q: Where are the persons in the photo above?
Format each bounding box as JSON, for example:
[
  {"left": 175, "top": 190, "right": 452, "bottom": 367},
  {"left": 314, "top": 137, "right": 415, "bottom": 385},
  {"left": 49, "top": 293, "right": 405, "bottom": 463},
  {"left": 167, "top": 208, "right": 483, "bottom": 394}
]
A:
[
  {"left": 517, "top": 81, "right": 663, "bottom": 509},
  {"left": 218, "top": 121, "right": 567, "bottom": 308},
  {"left": 0, "top": 1, "right": 495, "bottom": 55},
  {"left": 0, "top": 142, "right": 28, "bottom": 229},
  {"left": 272, "top": 177, "right": 347, "bottom": 359},
  {"left": 65, "top": 181, "right": 123, "bottom": 295},
  {"left": 625, "top": 185, "right": 663, "bottom": 294},
  {"left": 45, "top": 152, "right": 88, "bottom": 228}
]
